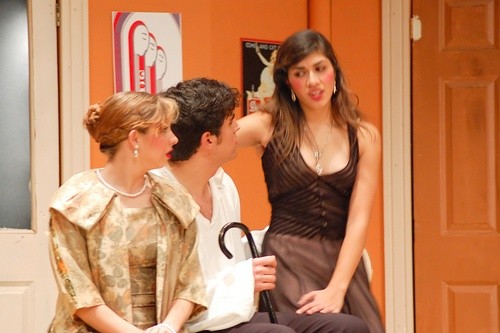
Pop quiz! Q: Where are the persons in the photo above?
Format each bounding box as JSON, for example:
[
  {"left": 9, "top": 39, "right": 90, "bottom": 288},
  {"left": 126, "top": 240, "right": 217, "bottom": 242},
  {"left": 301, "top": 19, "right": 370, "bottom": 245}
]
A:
[
  {"left": 234, "top": 28, "right": 385, "bottom": 333},
  {"left": 148, "top": 77, "right": 370, "bottom": 333},
  {"left": 47, "top": 91, "right": 208, "bottom": 333}
]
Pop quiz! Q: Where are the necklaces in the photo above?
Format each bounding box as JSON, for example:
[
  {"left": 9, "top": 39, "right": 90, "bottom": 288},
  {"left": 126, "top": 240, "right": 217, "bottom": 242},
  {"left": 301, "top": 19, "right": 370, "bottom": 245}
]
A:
[
  {"left": 303, "top": 127, "right": 322, "bottom": 175},
  {"left": 97, "top": 169, "right": 148, "bottom": 198}
]
